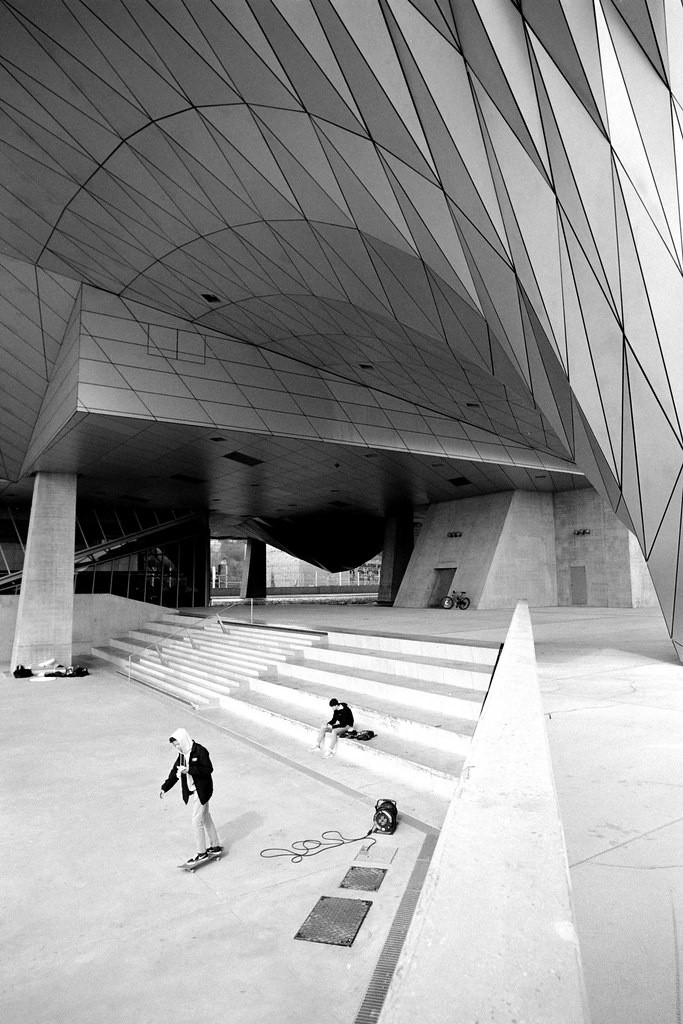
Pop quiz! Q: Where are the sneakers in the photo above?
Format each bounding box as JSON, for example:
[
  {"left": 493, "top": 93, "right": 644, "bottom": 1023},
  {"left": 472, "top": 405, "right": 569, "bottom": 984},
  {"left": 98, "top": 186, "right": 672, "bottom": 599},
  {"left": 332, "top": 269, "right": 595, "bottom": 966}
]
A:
[
  {"left": 309, "top": 744, "right": 321, "bottom": 751},
  {"left": 206, "top": 847, "right": 222, "bottom": 854},
  {"left": 186, "top": 852, "right": 210, "bottom": 864},
  {"left": 323, "top": 750, "right": 333, "bottom": 758}
]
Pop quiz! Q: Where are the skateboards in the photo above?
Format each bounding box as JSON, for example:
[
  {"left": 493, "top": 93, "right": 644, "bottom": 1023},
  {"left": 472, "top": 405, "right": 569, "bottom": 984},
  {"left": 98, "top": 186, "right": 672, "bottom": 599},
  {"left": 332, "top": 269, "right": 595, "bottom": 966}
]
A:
[{"left": 177, "top": 846, "right": 224, "bottom": 873}]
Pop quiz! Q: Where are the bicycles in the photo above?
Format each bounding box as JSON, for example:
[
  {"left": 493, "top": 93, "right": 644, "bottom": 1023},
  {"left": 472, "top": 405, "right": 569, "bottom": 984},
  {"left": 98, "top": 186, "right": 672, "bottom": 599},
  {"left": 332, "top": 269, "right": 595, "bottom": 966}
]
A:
[{"left": 439, "top": 590, "right": 471, "bottom": 610}]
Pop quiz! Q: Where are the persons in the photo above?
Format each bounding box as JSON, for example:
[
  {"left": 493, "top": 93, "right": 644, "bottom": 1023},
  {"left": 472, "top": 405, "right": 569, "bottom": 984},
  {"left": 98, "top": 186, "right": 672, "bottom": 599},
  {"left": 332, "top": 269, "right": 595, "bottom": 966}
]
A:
[
  {"left": 308, "top": 699, "right": 354, "bottom": 757},
  {"left": 159, "top": 727, "right": 222, "bottom": 867}
]
